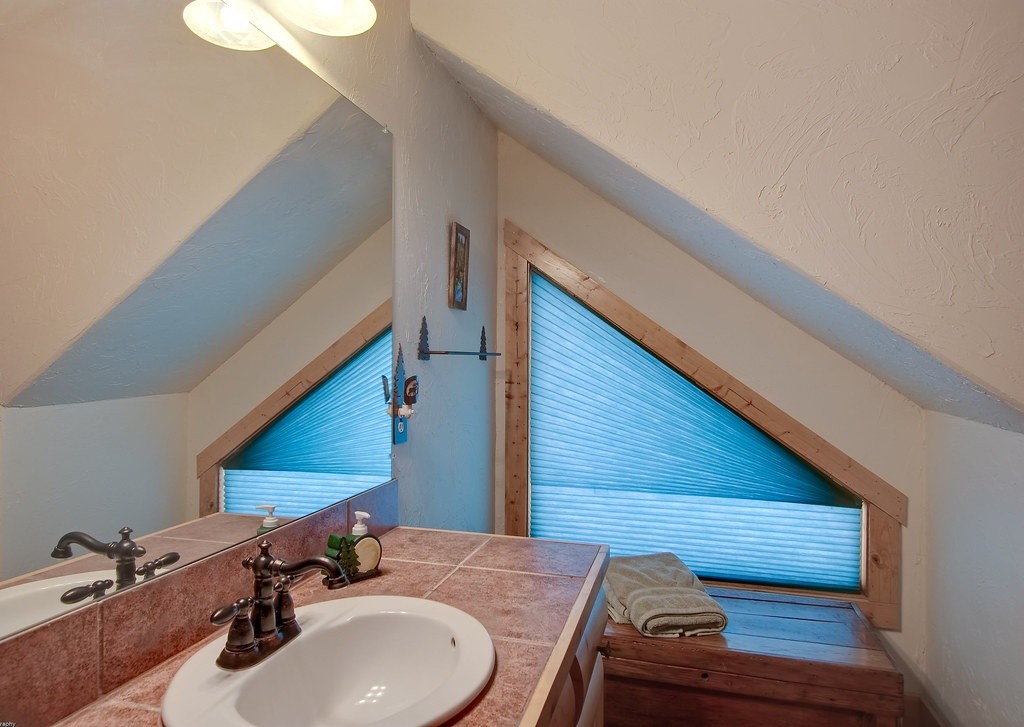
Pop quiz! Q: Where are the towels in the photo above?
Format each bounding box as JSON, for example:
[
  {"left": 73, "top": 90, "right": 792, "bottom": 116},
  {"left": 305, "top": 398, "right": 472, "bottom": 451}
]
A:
[{"left": 602, "top": 550, "right": 729, "bottom": 639}]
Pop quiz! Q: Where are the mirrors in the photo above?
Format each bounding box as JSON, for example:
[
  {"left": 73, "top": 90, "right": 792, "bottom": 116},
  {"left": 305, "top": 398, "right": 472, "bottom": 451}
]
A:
[{"left": 0, "top": 0, "right": 402, "bottom": 640}]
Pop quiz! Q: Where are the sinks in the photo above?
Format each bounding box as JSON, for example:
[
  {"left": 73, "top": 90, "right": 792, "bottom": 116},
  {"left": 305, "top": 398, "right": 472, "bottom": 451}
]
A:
[
  {"left": 0, "top": 578, "right": 150, "bottom": 640},
  {"left": 231, "top": 605, "right": 473, "bottom": 727}
]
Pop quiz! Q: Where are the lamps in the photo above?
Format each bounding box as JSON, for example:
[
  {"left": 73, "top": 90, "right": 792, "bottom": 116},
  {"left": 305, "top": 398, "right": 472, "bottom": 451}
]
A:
[
  {"left": 183, "top": 0, "right": 276, "bottom": 51},
  {"left": 276, "top": 0, "right": 379, "bottom": 38}
]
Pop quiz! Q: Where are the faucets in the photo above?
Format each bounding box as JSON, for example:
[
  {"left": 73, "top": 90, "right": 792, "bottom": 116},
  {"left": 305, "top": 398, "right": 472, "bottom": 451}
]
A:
[
  {"left": 50, "top": 524, "right": 148, "bottom": 593},
  {"left": 241, "top": 538, "right": 350, "bottom": 640}
]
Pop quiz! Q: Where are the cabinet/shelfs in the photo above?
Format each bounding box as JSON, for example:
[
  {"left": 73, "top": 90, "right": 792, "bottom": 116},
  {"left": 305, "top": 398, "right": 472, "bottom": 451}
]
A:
[{"left": 550, "top": 586, "right": 606, "bottom": 727}]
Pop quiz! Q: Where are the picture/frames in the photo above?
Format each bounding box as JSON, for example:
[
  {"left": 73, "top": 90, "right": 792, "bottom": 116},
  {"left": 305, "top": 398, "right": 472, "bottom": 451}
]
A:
[{"left": 447, "top": 222, "right": 470, "bottom": 312}]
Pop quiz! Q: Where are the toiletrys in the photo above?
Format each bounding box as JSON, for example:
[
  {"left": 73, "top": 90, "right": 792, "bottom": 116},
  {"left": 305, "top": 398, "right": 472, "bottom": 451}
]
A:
[
  {"left": 345, "top": 511, "right": 383, "bottom": 574},
  {"left": 255, "top": 504, "right": 281, "bottom": 536}
]
